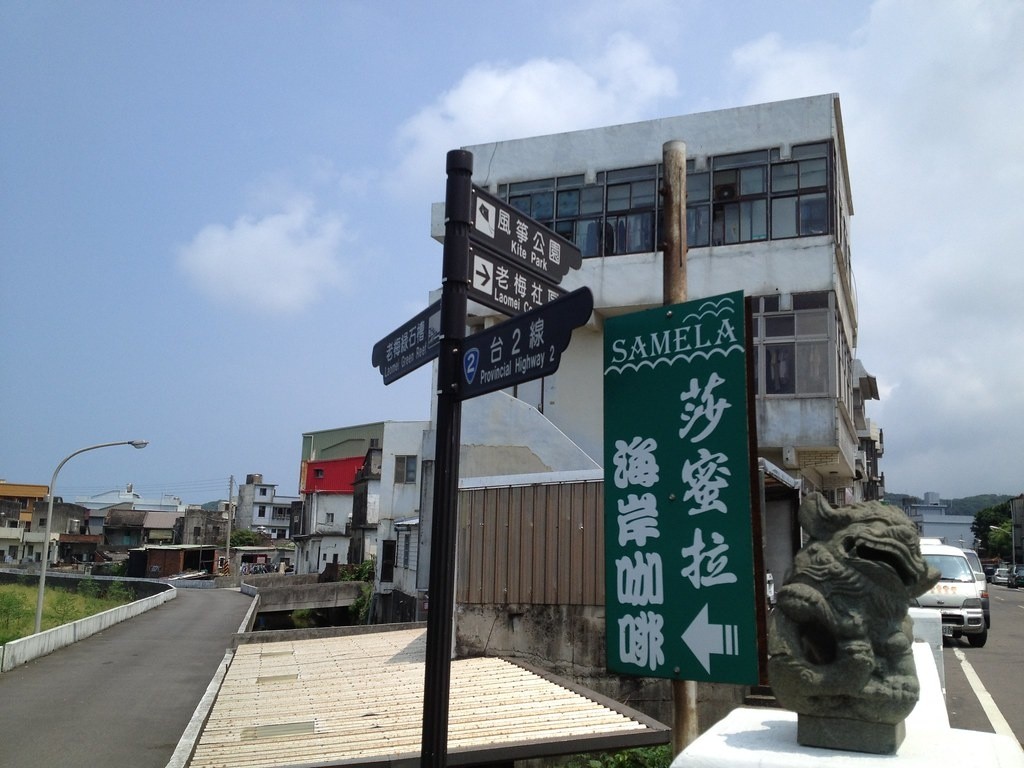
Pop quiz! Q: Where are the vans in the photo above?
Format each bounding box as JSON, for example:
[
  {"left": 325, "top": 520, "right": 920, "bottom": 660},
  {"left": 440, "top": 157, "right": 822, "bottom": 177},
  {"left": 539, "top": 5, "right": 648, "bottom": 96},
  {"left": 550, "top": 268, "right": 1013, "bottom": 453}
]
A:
[
  {"left": 958, "top": 548, "right": 990, "bottom": 628},
  {"left": 910, "top": 544, "right": 987, "bottom": 646}
]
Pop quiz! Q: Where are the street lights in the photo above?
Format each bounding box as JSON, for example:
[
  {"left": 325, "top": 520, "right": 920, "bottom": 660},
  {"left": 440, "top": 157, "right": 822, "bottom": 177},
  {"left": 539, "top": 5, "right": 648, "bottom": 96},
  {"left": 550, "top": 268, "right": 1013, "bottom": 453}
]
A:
[
  {"left": 990, "top": 526, "right": 1015, "bottom": 573},
  {"left": 35, "top": 439, "right": 148, "bottom": 634}
]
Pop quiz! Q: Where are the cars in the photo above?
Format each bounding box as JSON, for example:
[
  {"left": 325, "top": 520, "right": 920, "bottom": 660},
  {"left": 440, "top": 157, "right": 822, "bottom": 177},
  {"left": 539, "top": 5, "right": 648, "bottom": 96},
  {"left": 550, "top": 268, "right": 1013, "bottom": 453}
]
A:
[
  {"left": 984, "top": 567, "right": 995, "bottom": 577},
  {"left": 991, "top": 568, "right": 1011, "bottom": 584},
  {"left": 1008, "top": 565, "right": 1024, "bottom": 588}
]
